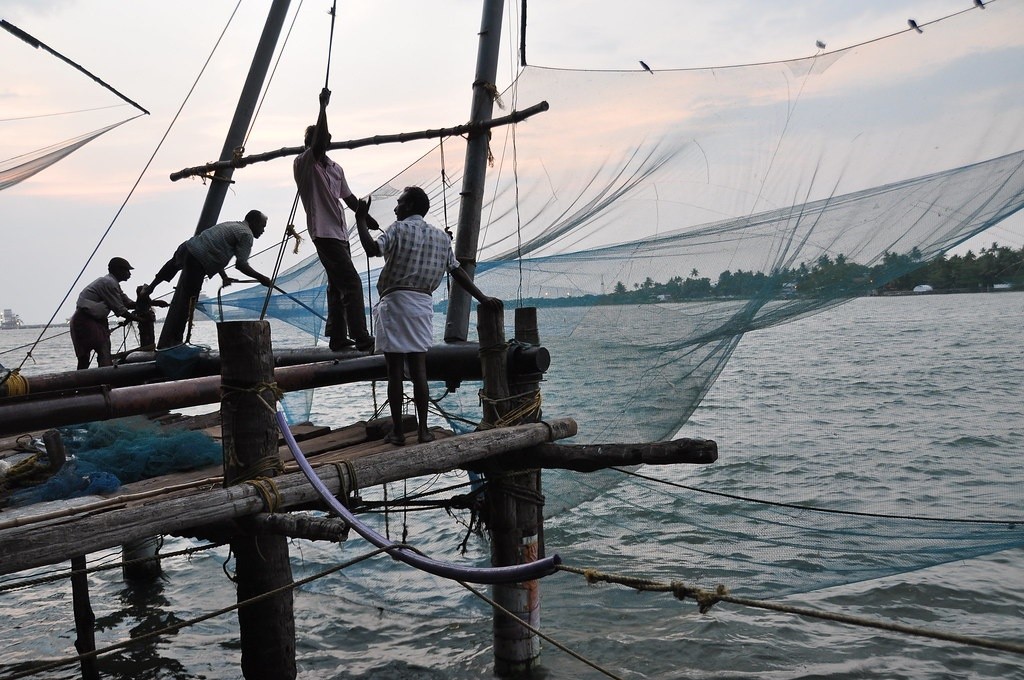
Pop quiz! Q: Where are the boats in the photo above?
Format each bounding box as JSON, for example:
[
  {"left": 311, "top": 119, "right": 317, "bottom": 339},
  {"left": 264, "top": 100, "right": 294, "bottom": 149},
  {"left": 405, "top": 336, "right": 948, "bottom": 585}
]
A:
[{"left": 0, "top": 309, "right": 21, "bottom": 329}]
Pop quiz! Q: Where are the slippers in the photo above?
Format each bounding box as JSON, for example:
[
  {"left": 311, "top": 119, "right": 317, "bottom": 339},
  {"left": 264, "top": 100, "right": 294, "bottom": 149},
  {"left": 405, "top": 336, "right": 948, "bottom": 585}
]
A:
[
  {"left": 418, "top": 428, "right": 435, "bottom": 444},
  {"left": 384, "top": 431, "right": 406, "bottom": 446}
]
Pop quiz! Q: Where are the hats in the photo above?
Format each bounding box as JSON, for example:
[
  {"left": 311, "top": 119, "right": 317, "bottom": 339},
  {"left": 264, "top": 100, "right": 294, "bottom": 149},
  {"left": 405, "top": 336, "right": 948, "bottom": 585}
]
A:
[{"left": 109, "top": 258, "right": 134, "bottom": 270}]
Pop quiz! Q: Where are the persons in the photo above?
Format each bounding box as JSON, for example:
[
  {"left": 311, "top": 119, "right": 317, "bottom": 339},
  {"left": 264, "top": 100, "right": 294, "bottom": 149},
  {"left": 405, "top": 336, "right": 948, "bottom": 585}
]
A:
[
  {"left": 144, "top": 211, "right": 271, "bottom": 349},
  {"left": 294, "top": 87, "right": 380, "bottom": 352},
  {"left": 70, "top": 257, "right": 168, "bottom": 370},
  {"left": 355, "top": 187, "right": 504, "bottom": 446}
]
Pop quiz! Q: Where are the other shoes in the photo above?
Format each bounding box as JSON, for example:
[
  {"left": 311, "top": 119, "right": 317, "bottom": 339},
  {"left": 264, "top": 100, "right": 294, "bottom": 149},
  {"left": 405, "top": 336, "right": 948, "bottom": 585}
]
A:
[
  {"left": 329, "top": 337, "right": 356, "bottom": 352},
  {"left": 356, "top": 335, "right": 375, "bottom": 352}
]
